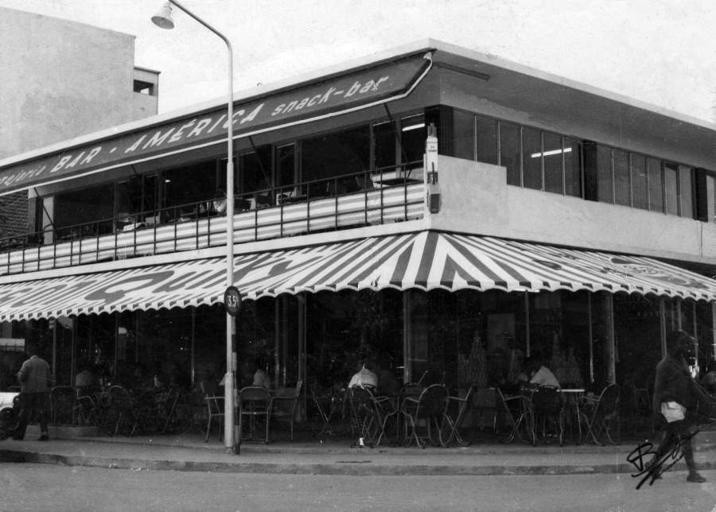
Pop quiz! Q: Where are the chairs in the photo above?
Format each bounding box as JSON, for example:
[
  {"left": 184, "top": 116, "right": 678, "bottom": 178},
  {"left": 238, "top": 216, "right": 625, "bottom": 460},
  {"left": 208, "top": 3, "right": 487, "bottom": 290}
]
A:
[
  {"left": 49, "top": 379, "right": 620, "bottom": 449},
  {"left": 354, "top": 174, "right": 373, "bottom": 189},
  {"left": 326, "top": 181, "right": 346, "bottom": 195}
]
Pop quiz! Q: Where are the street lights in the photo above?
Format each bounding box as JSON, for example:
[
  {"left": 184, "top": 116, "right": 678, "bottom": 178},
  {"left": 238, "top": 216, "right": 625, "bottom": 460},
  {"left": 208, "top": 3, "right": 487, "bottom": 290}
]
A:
[{"left": 152, "top": 0, "right": 235, "bottom": 454}]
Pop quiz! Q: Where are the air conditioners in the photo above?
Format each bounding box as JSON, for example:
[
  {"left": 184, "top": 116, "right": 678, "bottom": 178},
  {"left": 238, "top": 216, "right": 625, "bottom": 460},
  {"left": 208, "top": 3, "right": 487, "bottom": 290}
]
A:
[
  {"left": 242, "top": 198, "right": 256, "bottom": 211},
  {"left": 275, "top": 191, "right": 291, "bottom": 206}
]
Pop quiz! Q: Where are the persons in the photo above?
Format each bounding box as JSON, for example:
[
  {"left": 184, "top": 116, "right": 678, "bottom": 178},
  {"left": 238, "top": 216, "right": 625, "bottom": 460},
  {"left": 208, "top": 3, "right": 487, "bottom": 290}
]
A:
[
  {"left": 645, "top": 325, "right": 716, "bottom": 484},
  {"left": 6, "top": 340, "right": 51, "bottom": 441}
]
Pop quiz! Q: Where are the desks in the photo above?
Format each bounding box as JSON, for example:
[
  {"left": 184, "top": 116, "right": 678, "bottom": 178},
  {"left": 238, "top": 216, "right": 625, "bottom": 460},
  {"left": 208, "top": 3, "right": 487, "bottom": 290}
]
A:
[
  {"left": 278, "top": 192, "right": 330, "bottom": 202},
  {"left": 180, "top": 209, "right": 217, "bottom": 219},
  {"left": 374, "top": 178, "right": 423, "bottom": 185}
]
